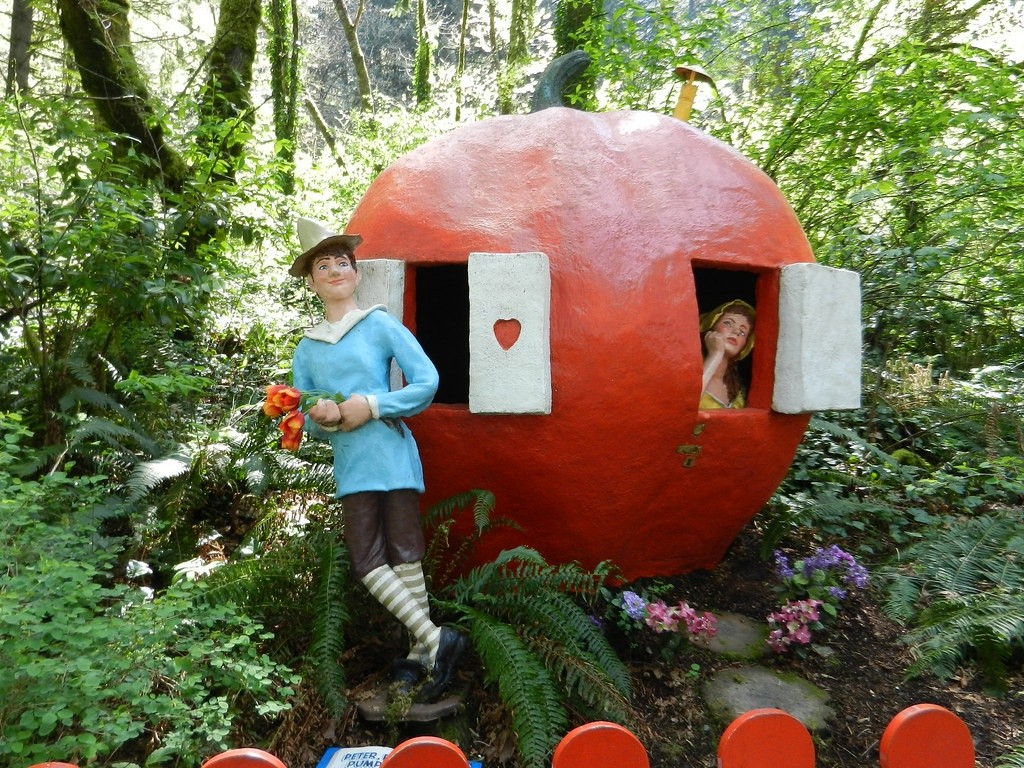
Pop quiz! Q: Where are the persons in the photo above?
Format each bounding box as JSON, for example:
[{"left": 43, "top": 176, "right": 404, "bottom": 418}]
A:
[
  {"left": 696, "top": 298, "right": 755, "bottom": 409},
  {"left": 289, "top": 218, "right": 466, "bottom": 703}
]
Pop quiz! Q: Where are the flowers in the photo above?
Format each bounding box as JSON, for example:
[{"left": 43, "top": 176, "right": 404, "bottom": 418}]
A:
[{"left": 262, "top": 384, "right": 407, "bottom": 451}]
